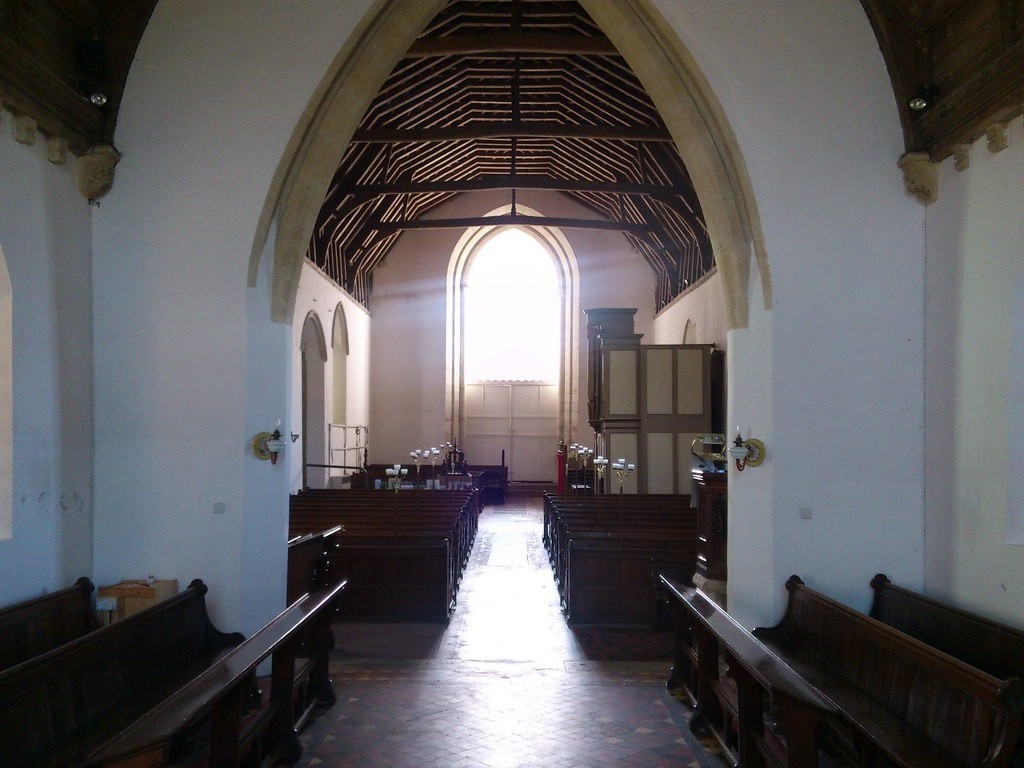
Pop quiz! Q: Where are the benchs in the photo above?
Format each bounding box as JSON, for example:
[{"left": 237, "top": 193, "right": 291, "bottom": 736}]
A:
[
  {"left": 0, "top": 576, "right": 262, "bottom": 768},
  {"left": 728, "top": 573, "right": 1024, "bottom": 768},
  {"left": 288, "top": 447, "right": 726, "bottom": 627}
]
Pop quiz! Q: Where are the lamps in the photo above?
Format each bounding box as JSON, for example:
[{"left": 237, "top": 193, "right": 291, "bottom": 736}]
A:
[
  {"left": 252, "top": 418, "right": 287, "bottom": 465},
  {"left": 728, "top": 424, "right": 766, "bottom": 472},
  {"left": 385, "top": 442, "right": 452, "bottom": 493},
  {"left": 570, "top": 443, "right": 635, "bottom": 495}
]
